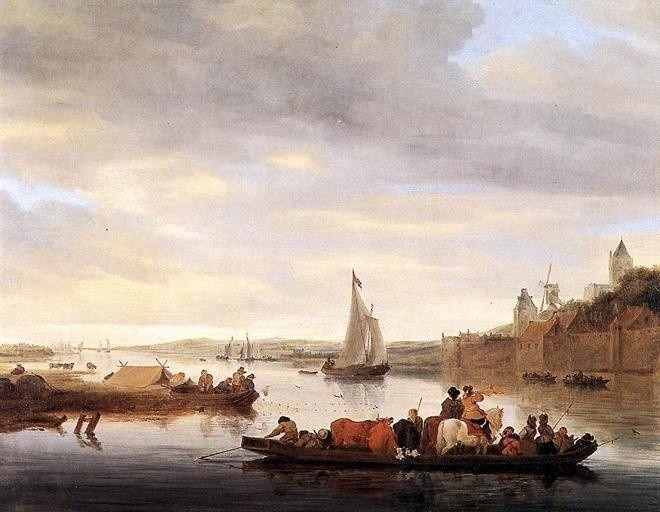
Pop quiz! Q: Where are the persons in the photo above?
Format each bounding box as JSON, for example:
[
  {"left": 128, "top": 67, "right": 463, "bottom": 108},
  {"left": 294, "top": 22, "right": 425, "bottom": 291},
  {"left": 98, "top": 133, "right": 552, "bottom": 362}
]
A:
[
  {"left": 406, "top": 408, "right": 423, "bottom": 453},
  {"left": 328, "top": 357, "right": 332, "bottom": 363},
  {"left": 197, "top": 366, "right": 257, "bottom": 395},
  {"left": 438, "top": 386, "right": 465, "bottom": 419},
  {"left": 538, "top": 412, "right": 557, "bottom": 454},
  {"left": 294, "top": 430, "right": 327, "bottom": 448},
  {"left": 497, "top": 425, "right": 523, "bottom": 457},
  {"left": 324, "top": 361, "right": 330, "bottom": 370},
  {"left": 461, "top": 384, "right": 494, "bottom": 444},
  {"left": 552, "top": 425, "right": 572, "bottom": 454},
  {"left": 10, "top": 363, "right": 26, "bottom": 376},
  {"left": 274, "top": 467, "right": 562, "bottom": 511},
  {"left": 264, "top": 415, "right": 298, "bottom": 445},
  {"left": 518, "top": 413, "right": 537, "bottom": 455},
  {"left": 330, "top": 359, "right": 336, "bottom": 367},
  {"left": 525, "top": 369, "right": 604, "bottom": 383}
]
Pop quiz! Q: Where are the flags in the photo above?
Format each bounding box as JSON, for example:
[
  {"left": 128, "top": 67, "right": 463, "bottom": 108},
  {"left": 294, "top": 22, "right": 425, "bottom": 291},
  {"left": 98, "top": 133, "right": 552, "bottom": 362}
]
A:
[{"left": 353, "top": 269, "right": 363, "bottom": 289}]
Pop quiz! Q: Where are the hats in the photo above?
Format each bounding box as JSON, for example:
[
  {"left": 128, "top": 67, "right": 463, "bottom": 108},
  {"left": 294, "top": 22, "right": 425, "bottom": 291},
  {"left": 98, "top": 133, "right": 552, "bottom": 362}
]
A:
[
  {"left": 248, "top": 374, "right": 255, "bottom": 379},
  {"left": 527, "top": 413, "right": 548, "bottom": 427},
  {"left": 237, "top": 367, "right": 246, "bottom": 373},
  {"left": 318, "top": 429, "right": 328, "bottom": 439},
  {"left": 277, "top": 416, "right": 290, "bottom": 424}
]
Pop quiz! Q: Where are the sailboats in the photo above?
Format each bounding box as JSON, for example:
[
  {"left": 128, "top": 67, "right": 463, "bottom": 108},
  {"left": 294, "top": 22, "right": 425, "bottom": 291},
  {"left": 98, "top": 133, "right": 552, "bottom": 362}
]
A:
[
  {"left": 323, "top": 269, "right": 392, "bottom": 381},
  {"left": 78, "top": 337, "right": 112, "bottom": 354},
  {"left": 214, "top": 328, "right": 275, "bottom": 363}
]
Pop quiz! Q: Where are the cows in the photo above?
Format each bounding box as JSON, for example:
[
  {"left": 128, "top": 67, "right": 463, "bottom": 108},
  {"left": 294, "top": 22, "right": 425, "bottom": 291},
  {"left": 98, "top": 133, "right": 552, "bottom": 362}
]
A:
[
  {"left": 63, "top": 363, "right": 75, "bottom": 371},
  {"left": 49, "top": 362, "right": 62, "bottom": 371},
  {"left": 10, "top": 363, "right": 25, "bottom": 374},
  {"left": 86, "top": 361, "right": 96, "bottom": 372},
  {"left": 330, "top": 416, "right": 396, "bottom": 456}
]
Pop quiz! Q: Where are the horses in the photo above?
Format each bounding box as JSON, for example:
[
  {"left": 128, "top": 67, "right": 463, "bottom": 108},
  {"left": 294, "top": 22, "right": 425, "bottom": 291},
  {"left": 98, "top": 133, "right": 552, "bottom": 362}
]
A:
[{"left": 422, "top": 406, "right": 505, "bottom": 455}]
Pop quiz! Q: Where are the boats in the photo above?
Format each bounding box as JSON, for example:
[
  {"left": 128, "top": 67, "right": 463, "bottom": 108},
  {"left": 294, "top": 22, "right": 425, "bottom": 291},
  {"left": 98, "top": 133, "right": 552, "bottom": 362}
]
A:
[
  {"left": 299, "top": 369, "right": 319, "bottom": 375},
  {"left": 522, "top": 371, "right": 557, "bottom": 385},
  {"left": 240, "top": 420, "right": 599, "bottom": 476},
  {"left": 564, "top": 371, "right": 611, "bottom": 389},
  {"left": 171, "top": 376, "right": 261, "bottom": 413}
]
